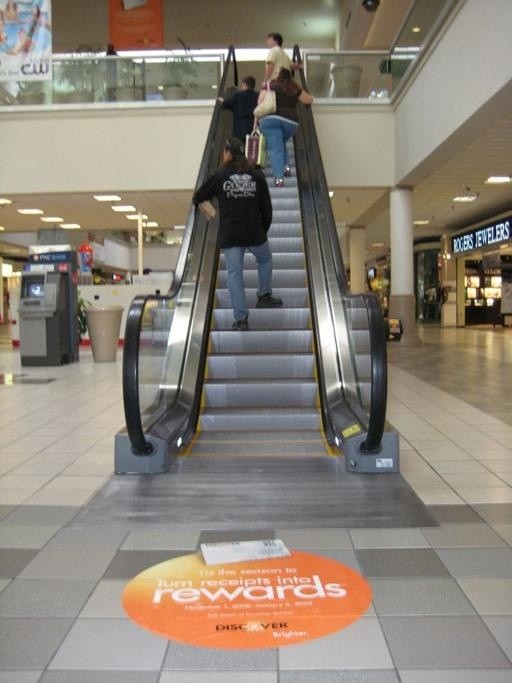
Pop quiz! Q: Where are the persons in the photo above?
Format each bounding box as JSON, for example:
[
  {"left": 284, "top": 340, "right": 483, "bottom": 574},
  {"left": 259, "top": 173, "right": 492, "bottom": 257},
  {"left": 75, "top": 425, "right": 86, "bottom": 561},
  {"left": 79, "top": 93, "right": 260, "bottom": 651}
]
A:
[
  {"left": 262, "top": 31, "right": 306, "bottom": 78},
  {"left": 260, "top": 64, "right": 313, "bottom": 188},
  {"left": 217, "top": 74, "right": 260, "bottom": 142},
  {"left": 192, "top": 133, "right": 283, "bottom": 331},
  {"left": 249, "top": 78, "right": 272, "bottom": 166}
]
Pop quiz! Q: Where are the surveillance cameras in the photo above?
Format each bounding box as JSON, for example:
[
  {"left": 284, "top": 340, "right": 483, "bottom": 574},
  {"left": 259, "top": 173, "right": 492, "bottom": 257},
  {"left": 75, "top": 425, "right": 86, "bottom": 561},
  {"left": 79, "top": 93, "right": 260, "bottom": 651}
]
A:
[{"left": 362, "top": 0, "right": 380, "bottom": 12}]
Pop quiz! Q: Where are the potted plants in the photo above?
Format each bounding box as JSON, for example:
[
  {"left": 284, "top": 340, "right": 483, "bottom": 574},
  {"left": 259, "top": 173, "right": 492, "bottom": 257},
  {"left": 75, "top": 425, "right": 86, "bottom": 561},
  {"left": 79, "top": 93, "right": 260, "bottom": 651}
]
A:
[{"left": 14, "top": 36, "right": 202, "bottom": 104}]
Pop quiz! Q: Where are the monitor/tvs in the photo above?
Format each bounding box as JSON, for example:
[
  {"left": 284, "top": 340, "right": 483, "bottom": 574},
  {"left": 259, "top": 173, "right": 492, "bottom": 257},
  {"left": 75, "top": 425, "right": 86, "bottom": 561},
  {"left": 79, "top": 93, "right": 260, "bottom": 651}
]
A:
[{"left": 26, "top": 282, "right": 43, "bottom": 297}]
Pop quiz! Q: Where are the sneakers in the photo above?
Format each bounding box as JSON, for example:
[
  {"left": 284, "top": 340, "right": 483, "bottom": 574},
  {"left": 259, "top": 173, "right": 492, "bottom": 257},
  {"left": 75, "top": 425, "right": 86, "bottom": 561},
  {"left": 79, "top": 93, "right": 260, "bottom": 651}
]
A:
[
  {"left": 256, "top": 295, "right": 283, "bottom": 307},
  {"left": 232, "top": 321, "right": 248, "bottom": 331}
]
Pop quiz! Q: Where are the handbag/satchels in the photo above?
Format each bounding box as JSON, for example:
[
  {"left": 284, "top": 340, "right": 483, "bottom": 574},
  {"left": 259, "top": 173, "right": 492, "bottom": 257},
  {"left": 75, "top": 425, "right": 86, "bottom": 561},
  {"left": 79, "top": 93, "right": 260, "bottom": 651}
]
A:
[
  {"left": 254, "top": 90, "right": 276, "bottom": 117},
  {"left": 246, "top": 134, "right": 266, "bottom": 168}
]
